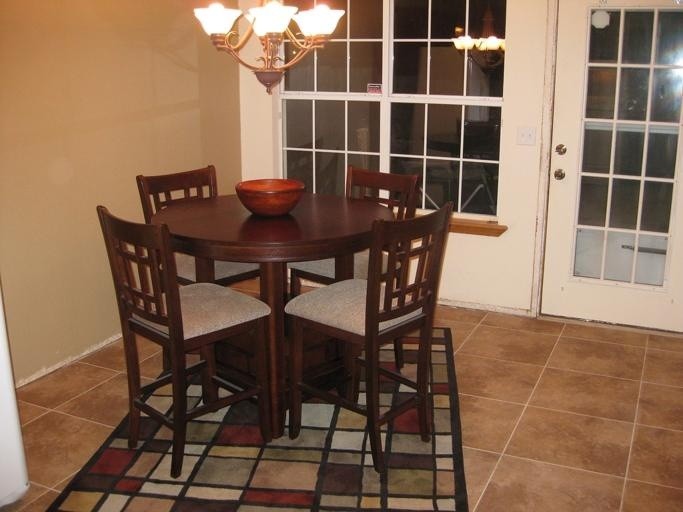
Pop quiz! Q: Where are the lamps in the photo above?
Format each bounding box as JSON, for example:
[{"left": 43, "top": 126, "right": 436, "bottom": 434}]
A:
[
  {"left": 444, "top": 0, "right": 508, "bottom": 74},
  {"left": 185, "top": 1, "right": 349, "bottom": 99}
]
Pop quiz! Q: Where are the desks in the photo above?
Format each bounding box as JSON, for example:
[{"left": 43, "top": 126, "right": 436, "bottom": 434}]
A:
[{"left": 147, "top": 189, "right": 402, "bottom": 441}]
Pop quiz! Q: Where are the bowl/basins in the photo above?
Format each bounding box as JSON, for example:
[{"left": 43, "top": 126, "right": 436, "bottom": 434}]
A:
[{"left": 233, "top": 178, "right": 305, "bottom": 217}]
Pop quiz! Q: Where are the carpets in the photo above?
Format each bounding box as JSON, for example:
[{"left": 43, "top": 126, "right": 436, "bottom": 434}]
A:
[{"left": 38, "top": 323, "right": 472, "bottom": 512}]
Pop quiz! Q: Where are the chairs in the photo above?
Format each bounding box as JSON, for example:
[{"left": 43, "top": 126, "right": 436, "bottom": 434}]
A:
[
  {"left": 137, "top": 165, "right": 227, "bottom": 376},
  {"left": 310, "top": 145, "right": 340, "bottom": 195},
  {"left": 393, "top": 116, "right": 500, "bottom": 214},
  {"left": 279, "top": 164, "right": 431, "bottom": 379},
  {"left": 285, "top": 131, "right": 325, "bottom": 191},
  {"left": 97, "top": 205, "right": 273, "bottom": 481},
  {"left": 281, "top": 198, "right": 461, "bottom": 478}
]
